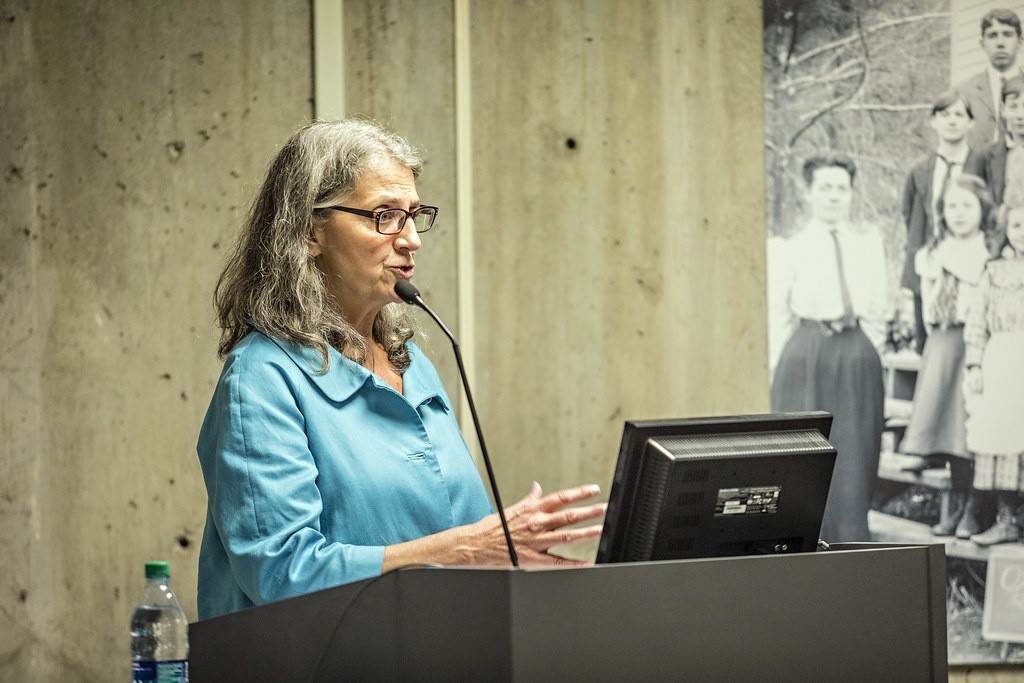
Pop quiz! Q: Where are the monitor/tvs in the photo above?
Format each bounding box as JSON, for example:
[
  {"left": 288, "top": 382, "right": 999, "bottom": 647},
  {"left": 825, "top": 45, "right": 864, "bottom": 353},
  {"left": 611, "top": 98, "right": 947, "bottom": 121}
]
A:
[{"left": 595, "top": 411, "right": 838, "bottom": 563}]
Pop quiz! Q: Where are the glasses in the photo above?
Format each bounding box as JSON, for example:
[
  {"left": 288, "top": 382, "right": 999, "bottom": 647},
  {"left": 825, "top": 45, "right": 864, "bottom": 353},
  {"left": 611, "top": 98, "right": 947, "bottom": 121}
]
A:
[{"left": 332, "top": 205, "right": 439, "bottom": 234}]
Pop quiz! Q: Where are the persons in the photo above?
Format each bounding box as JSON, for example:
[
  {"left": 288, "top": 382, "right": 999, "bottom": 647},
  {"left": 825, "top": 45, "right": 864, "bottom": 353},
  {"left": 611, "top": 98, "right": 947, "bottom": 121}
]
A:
[
  {"left": 197, "top": 118, "right": 610, "bottom": 625},
  {"left": 767, "top": 146, "right": 898, "bottom": 541},
  {"left": 890, "top": 7, "right": 1023, "bottom": 551}
]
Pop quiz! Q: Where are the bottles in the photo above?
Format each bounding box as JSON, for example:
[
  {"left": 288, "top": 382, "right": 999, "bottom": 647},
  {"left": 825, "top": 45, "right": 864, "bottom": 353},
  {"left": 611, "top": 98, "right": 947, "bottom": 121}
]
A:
[{"left": 129, "top": 560, "right": 190, "bottom": 683}]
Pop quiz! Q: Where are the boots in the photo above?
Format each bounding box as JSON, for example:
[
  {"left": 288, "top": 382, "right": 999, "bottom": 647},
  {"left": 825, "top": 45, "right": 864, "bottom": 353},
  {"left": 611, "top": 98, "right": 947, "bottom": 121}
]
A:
[
  {"left": 933, "top": 466, "right": 970, "bottom": 535},
  {"left": 956, "top": 487, "right": 994, "bottom": 538},
  {"left": 970, "top": 490, "right": 1020, "bottom": 545}
]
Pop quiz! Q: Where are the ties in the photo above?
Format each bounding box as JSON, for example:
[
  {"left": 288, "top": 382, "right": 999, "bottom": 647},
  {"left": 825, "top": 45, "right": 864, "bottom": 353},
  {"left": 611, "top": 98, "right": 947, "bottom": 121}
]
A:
[
  {"left": 831, "top": 229, "right": 854, "bottom": 328},
  {"left": 998, "top": 77, "right": 1006, "bottom": 142},
  {"left": 936, "top": 155, "right": 954, "bottom": 215}
]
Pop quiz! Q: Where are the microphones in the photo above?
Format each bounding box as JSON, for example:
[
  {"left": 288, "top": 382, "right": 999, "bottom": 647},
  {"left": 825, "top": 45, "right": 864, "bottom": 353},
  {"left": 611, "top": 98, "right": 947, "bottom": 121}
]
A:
[{"left": 393, "top": 280, "right": 520, "bottom": 568}]
